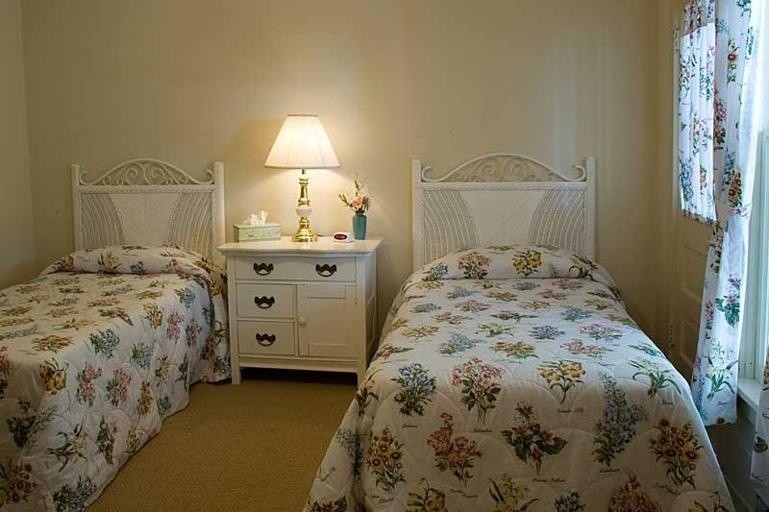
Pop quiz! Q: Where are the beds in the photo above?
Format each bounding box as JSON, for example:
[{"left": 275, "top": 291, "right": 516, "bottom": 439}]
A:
[
  {"left": 2, "top": 159, "right": 226, "bottom": 510},
  {"left": 363, "top": 153, "right": 687, "bottom": 511}
]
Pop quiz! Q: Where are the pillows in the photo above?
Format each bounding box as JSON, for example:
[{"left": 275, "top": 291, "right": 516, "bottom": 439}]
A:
[
  {"left": 71, "top": 246, "right": 187, "bottom": 273},
  {"left": 444, "top": 244, "right": 580, "bottom": 280}
]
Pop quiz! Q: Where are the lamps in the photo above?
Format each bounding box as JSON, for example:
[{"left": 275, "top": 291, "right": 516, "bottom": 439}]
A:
[{"left": 264, "top": 114, "right": 340, "bottom": 244}]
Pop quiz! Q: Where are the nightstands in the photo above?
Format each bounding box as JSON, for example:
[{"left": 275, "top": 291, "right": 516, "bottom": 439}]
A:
[{"left": 216, "top": 236, "right": 382, "bottom": 393}]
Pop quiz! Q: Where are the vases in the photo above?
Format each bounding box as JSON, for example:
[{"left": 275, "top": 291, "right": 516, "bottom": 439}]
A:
[{"left": 353, "top": 212, "right": 367, "bottom": 239}]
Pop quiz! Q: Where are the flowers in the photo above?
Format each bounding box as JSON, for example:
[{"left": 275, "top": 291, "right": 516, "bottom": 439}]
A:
[{"left": 337, "top": 180, "right": 371, "bottom": 211}]
